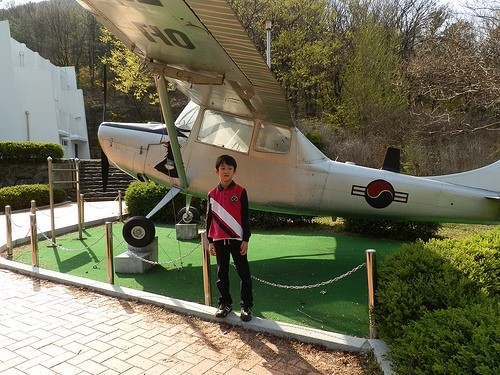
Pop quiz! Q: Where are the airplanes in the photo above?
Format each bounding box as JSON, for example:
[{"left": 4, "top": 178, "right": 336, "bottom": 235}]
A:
[{"left": 78, "top": 0, "right": 500, "bottom": 250}]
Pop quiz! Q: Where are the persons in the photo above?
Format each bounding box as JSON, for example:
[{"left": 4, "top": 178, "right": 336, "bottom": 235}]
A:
[{"left": 206, "top": 154, "right": 254, "bottom": 322}]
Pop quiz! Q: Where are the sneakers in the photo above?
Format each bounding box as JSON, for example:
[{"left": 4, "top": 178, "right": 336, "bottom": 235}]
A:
[
  {"left": 215, "top": 305, "right": 232, "bottom": 318},
  {"left": 240, "top": 308, "right": 253, "bottom": 322}
]
